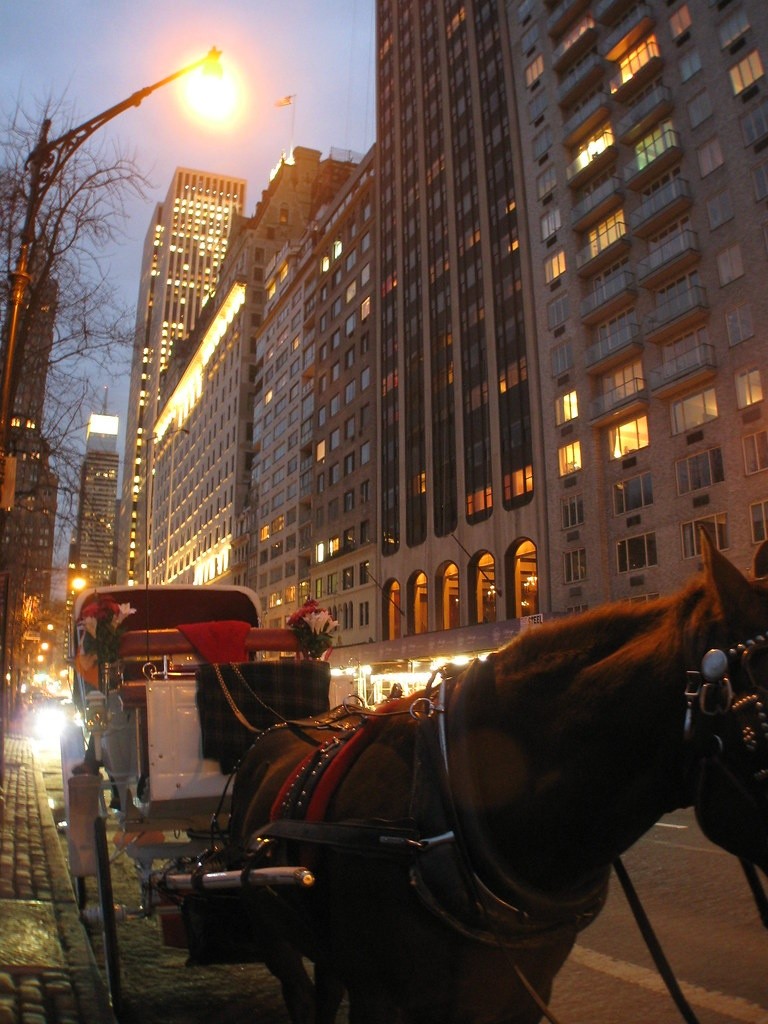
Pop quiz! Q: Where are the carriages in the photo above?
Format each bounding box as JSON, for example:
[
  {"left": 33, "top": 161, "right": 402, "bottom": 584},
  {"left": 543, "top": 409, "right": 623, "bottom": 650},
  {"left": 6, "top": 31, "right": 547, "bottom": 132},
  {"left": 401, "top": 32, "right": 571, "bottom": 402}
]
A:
[{"left": 63, "top": 528, "right": 767, "bottom": 1024}]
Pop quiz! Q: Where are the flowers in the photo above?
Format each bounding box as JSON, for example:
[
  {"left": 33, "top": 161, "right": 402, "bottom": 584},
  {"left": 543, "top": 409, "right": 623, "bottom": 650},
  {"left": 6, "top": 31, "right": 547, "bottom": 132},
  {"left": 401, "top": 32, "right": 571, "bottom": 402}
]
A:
[
  {"left": 76, "top": 592, "right": 137, "bottom": 663},
  {"left": 288, "top": 597, "right": 341, "bottom": 660}
]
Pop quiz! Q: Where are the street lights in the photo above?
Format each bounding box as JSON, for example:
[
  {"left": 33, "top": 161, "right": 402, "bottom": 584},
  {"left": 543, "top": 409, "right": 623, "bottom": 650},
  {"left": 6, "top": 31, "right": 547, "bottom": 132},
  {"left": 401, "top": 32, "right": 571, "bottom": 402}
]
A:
[
  {"left": 9, "top": 558, "right": 87, "bottom": 719},
  {"left": 0, "top": 46, "right": 241, "bottom": 489}
]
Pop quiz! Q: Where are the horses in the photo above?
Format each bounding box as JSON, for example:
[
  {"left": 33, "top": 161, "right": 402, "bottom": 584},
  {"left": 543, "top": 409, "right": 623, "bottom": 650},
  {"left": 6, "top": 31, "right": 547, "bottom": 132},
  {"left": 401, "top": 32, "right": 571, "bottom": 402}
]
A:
[{"left": 224, "top": 522, "right": 768, "bottom": 1024}]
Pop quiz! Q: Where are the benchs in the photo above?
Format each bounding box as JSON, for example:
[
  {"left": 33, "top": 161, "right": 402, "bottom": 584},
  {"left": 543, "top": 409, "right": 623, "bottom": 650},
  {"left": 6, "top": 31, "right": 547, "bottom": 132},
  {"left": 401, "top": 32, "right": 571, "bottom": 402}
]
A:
[{"left": 116, "top": 624, "right": 331, "bottom": 699}]
